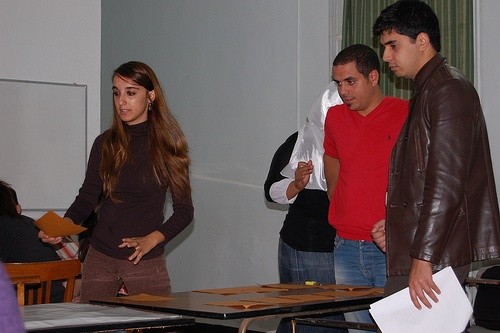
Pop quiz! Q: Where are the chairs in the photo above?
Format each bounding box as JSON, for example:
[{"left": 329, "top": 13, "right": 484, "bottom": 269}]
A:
[{"left": 0, "top": 259, "right": 82, "bottom": 307}]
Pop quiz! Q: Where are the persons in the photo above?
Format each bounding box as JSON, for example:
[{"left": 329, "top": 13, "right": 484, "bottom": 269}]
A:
[
  {"left": 264, "top": 79, "right": 345, "bottom": 333},
  {"left": 37, "top": 60, "right": 195, "bottom": 304},
  {"left": 372, "top": 0, "right": 500, "bottom": 333},
  {"left": 0, "top": 181, "right": 129, "bottom": 303},
  {"left": 324, "top": 44, "right": 412, "bottom": 333}
]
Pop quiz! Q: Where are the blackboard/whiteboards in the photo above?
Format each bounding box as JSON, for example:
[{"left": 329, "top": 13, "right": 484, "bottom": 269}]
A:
[{"left": 0, "top": 78, "right": 87, "bottom": 211}]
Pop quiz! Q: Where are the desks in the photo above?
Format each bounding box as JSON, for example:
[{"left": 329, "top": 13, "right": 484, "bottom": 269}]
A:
[
  {"left": 89, "top": 279, "right": 389, "bottom": 333},
  {"left": 17, "top": 300, "right": 197, "bottom": 333}
]
